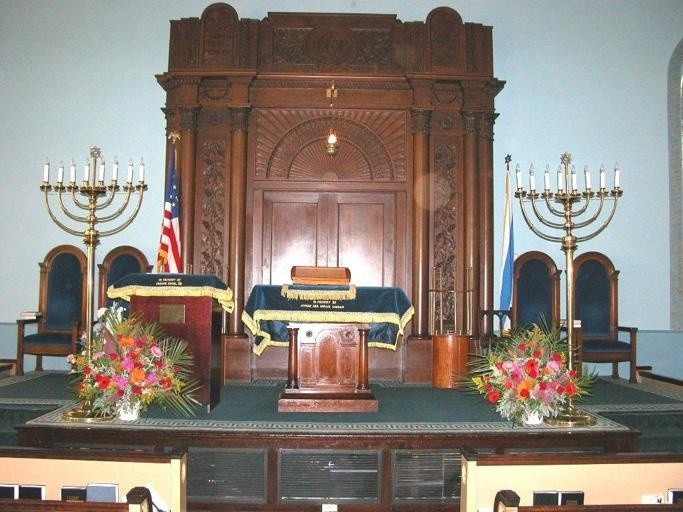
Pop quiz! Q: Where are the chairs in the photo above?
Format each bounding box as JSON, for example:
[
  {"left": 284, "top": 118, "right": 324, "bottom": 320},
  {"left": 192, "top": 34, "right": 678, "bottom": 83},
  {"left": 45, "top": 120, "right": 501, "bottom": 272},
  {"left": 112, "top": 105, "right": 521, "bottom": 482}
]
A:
[
  {"left": 513, "top": 250, "right": 561, "bottom": 333},
  {"left": 569, "top": 251, "right": 638, "bottom": 383},
  {"left": 17, "top": 244, "right": 88, "bottom": 376},
  {"left": 97, "top": 244, "right": 154, "bottom": 311}
]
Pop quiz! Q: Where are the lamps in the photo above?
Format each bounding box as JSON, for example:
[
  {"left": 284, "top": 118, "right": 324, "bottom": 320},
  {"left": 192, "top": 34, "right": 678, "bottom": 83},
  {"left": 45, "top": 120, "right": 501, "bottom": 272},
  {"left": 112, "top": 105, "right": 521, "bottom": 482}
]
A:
[{"left": 326, "top": 131, "right": 338, "bottom": 155}]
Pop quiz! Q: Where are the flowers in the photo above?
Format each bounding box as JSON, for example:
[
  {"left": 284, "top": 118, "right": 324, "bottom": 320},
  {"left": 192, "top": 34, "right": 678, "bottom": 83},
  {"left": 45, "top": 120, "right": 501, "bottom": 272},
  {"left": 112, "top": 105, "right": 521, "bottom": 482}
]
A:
[
  {"left": 451, "top": 312, "right": 599, "bottom": 427},
  {"left": 65, "top": 301, "right": 205, "bottom": 422}
]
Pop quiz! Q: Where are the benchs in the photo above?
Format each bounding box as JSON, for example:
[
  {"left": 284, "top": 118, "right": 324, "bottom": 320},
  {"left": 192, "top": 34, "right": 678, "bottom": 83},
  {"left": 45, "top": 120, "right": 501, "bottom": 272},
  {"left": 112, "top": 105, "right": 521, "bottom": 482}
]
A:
[
  {"left": 0, "top": 445, "right": 188, "bottom": 511},
  {"left": 459, "top": 445, "right": 682, "bottom": 511}
]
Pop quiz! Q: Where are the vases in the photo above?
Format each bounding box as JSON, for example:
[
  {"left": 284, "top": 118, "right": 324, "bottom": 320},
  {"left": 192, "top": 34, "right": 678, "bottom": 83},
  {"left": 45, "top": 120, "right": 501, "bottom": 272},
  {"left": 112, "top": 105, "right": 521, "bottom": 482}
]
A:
[
  {"left": 116, "top": 398, "right": 141, "bottom": 422},
  {"left": 522, "top": 412, "right": 542, "bottom": 426}
]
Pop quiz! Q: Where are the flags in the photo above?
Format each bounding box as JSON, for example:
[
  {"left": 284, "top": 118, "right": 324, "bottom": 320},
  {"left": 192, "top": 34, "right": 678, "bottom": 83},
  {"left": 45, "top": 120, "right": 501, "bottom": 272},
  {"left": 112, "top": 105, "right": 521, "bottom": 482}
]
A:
[
  {"left": 500, "top": 171, "right": 515, "bottom": 336},
  {"left": 158, "top": 143, "right": 184, "bottom": 273}
]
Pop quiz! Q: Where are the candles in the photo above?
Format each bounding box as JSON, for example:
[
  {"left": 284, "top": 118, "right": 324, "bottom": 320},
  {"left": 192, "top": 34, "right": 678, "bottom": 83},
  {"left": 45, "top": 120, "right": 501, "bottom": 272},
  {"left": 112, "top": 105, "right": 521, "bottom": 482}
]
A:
[
  {"left": 516, "top": 166, "right": 621, "bottom": 190},
  {"left": 42, "top": 156, "right": 145, "bottom": 183}
]
